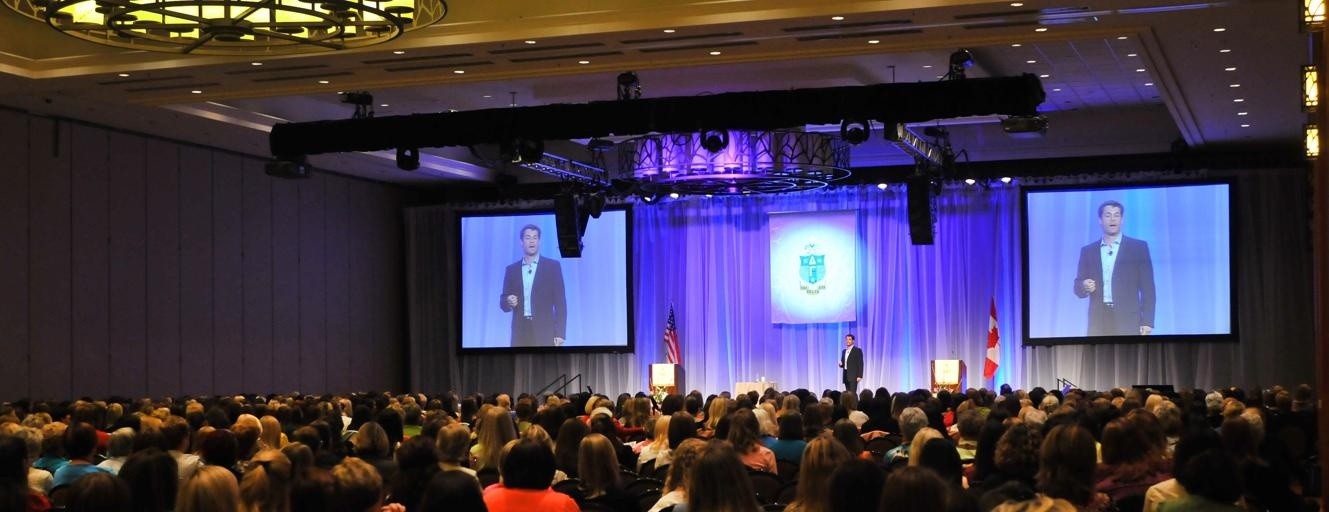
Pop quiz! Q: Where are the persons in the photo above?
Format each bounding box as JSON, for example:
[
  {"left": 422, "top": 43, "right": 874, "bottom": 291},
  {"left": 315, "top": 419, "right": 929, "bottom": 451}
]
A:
[
  {"left": 1072, "top": 200, "right": 1155, "bottom": 337},
  {"left": 0, "top": 383, "right": 1329, "bottom": 512},
  {"left": 500, "top": 224, "right": 567, "bottom": 347},
  {"left": 838, "top": 334, "right": 863, "bottom": 394}
]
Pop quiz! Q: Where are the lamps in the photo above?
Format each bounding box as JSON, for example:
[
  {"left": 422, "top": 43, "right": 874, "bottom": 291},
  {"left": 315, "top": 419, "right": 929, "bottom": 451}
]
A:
[
  {"left": 1296, "top": 0, "right": 1325, "bottom": 162},
  {"left": 267, "top": 156, "right": 309, "bottom": 182},
  {"left": 1004, "top": 115, "right": 1049, "bottom": 136},
  {"left": 341, "top": 91, "right": 375, "bottom": 114}
]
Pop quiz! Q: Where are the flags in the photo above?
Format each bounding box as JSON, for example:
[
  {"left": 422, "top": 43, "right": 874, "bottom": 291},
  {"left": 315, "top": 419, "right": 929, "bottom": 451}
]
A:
[
  {"left": 663, "top": 303, "right": 682, "bottom": 365},
  {"left": 984, "top": 295, "right": 1000, "bottom": 381}
]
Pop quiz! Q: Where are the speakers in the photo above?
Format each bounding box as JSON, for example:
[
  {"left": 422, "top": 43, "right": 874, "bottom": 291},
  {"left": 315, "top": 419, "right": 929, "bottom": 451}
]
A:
[
  {"left": 553, "top": 182, "right": 584, "bottom": 258},
  {"left": 907, "top": 177, "right": 934, "bottom": 245}
]
